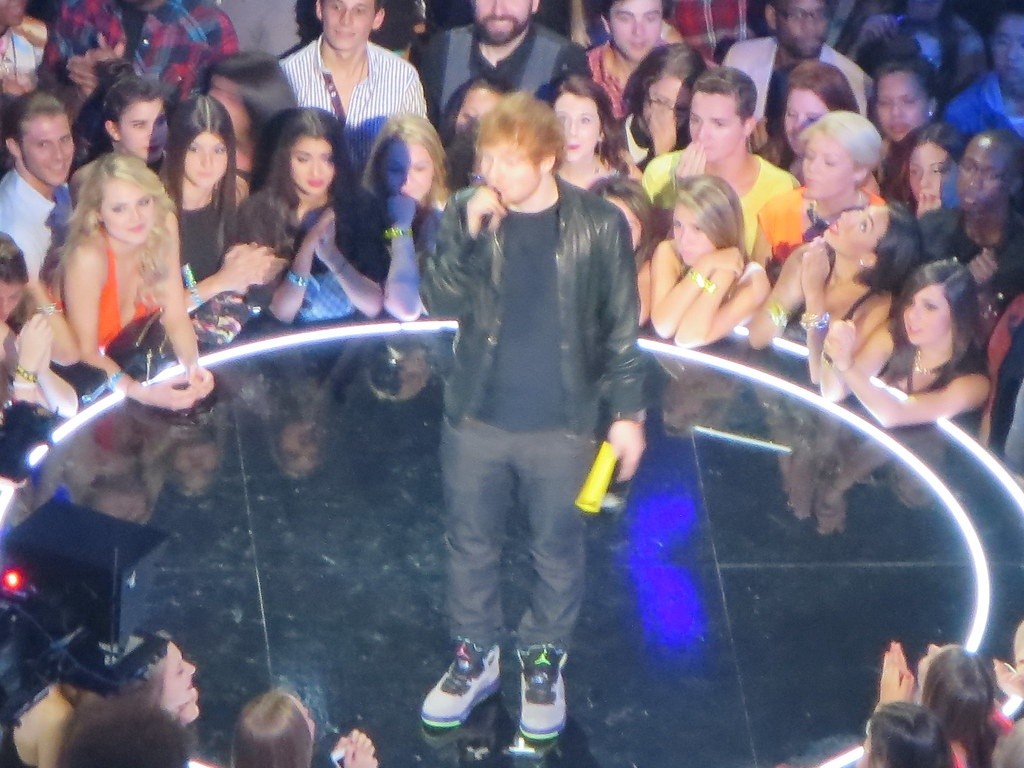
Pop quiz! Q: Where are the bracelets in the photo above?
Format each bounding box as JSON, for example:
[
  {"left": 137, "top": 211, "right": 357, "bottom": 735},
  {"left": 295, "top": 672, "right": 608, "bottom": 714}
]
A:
[
  {"left": 107, "top": 368, "right": 125, "bottom": 390},
  {"left": 189, "top": 284, "right": 204, "bottom": 308},
  {"left": 762, "top": 296, "right": 791, "bottom": 328},
  {"left": 13, "top": 381, "right": 38, "bottom": 389},
  {"left": 384, "top": 227, "right": 413, "bottom": 236},
  {"left": 331, "top": 258, "right": 350, "bottom": 276},
  {"left": 836, "top": 354, "right": 854, "bottom": 371},
  {"left": 688, "top": 266, "right": 707, "bottom": 289},
  {"left": 799, "top": 312, "right": 830, "bottom": 331},
  {"left": 820, "top": 353, "right": 833, "bottom": 369},
  {"left": 16, "top": 362, "right": 37, "bottom": 383},
  {"left": 703, "top": 278, "right": 724, "bottom": 298}
]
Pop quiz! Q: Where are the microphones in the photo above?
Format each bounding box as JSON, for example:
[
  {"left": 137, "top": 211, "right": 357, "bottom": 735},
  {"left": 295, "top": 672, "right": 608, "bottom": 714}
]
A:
[{"left": 480, "top": 189, "right": 501, "bottom": 232}]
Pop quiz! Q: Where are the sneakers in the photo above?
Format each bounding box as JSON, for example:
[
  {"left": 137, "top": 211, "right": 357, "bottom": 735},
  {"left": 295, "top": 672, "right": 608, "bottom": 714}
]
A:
[
  {"left": 421, "top": 637, "right": 502, "bottom": 727},
  {"left": 518, "top": 642, "right": 568, "bottom": 741}
]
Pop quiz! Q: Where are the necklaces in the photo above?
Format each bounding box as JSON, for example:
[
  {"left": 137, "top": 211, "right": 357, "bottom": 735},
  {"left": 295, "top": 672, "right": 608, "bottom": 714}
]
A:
[
  {"left": 320, "top": 50, "right": 365, "bottom": 84},
  {"left": 914, "top": 346, "right": 951, "bottom": 377}
]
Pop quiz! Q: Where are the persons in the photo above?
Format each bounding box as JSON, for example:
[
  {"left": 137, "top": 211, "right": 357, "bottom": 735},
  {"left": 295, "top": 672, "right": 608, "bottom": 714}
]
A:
[
  {"left": 0, "top": 636, "right": 379, "bottom": 768},
  {"left": 0, "top": 0, "right": 1024, "bottom": 473},
  {"left": 9, "top": 330, "right": 433, "bottom": 526},
  {"left": 419, "top": 92, "right": 646, "bottom": 739},
  {"left": 855, "top": 622, "right": 1024, "bottom": 768},
  {"left": 652, "top": 352, "right": 948, "bottom": 536}
]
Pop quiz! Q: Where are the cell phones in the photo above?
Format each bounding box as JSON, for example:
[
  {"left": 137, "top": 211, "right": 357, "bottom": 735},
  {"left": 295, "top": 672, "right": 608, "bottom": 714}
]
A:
[{"left": 329, "top": 747, "right": 344, "bottom": 768}]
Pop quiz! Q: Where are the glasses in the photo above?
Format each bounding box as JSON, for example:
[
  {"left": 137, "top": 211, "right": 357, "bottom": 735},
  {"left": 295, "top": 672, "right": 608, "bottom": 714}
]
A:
[
  {"left": 775, "top": 7, "right": 832, "bottom": 22},
  {"left": 957, "top": 160, "right": 1013, "bottom": 186},
  {"left": 646, "top": 90, "right": 689, "bottom": 117}
]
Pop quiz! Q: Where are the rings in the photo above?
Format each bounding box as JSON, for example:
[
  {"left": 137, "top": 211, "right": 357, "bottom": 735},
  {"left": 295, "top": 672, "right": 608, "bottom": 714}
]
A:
[{"left": 818, "top": 248, "right": 825, "bottom": 253}]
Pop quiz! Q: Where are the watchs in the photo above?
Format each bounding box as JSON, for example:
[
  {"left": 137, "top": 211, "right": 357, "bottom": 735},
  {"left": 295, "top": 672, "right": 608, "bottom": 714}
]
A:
[
  {"left": 286, "top": 269, "right": 310, "bottom": 287},
  {"left": 611, "top": 409, "right": 644, "bottom": 425}
]
